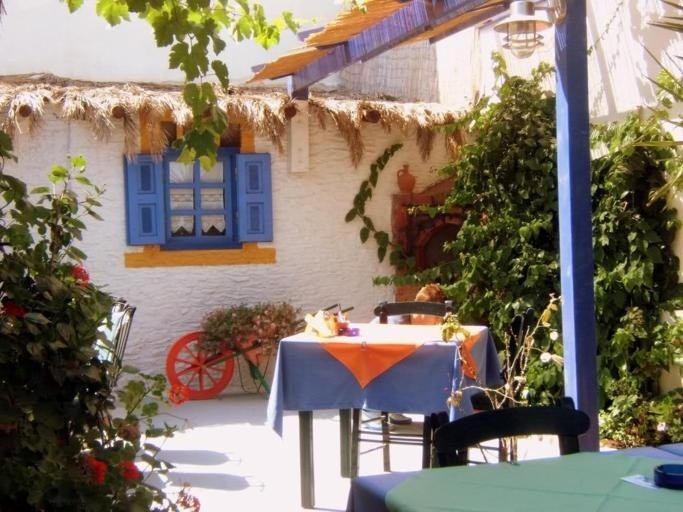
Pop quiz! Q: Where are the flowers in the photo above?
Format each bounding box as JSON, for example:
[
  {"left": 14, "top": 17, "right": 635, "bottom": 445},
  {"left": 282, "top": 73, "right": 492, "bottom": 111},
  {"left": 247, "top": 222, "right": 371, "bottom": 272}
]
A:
[
  {"left": 202, "top": 299, "right": 301, "bottom": 355},
  {"left": 0, "top": 130, "right": 200, "bottom": 511}
]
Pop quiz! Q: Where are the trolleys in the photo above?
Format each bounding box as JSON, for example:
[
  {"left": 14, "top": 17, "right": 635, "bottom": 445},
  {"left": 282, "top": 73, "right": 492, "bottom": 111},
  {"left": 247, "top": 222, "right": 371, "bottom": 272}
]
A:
[{"left": 167, "top": 299, "right": 354, "bottom": 402}]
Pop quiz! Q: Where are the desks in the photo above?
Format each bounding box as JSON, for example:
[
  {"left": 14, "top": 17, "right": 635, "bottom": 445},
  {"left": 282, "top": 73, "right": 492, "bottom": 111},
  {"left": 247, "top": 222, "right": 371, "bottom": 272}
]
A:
[
  {"left": 270, "top": 321, "right": 506, "bottom": 509},
  {"left": 93, "top": 313, "right": 111, "bottom": 363},
  {"left": 342, "top": 442, "right": 682, "bottom": 512}
]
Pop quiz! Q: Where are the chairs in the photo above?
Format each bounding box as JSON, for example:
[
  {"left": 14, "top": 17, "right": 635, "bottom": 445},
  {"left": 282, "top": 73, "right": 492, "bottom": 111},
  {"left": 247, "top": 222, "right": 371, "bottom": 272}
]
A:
[
  {"left": 102, "top": 304, "right": 135, "bottom": 382},
  {"left": 432, "top": 396, "right": 591, "bottom": 468},
  {"left": 100, "top": 296, "right": 125, "bottom": 364},
  {"left": 349, "top": 297, "right": 453, "bottom": 473}
]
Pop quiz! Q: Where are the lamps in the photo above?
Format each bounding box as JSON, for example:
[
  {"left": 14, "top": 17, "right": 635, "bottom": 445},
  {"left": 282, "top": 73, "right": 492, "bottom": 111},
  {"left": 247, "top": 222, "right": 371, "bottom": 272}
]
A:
[{"left": 478, "top": 0, "right": 553, "bottom": 59}]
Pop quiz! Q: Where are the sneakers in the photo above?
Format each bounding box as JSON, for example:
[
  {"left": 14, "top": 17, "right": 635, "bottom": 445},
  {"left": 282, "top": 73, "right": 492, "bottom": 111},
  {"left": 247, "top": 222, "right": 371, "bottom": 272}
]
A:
[{"left": 388, "top": 413, "right": 412, "bottom": 425}]
